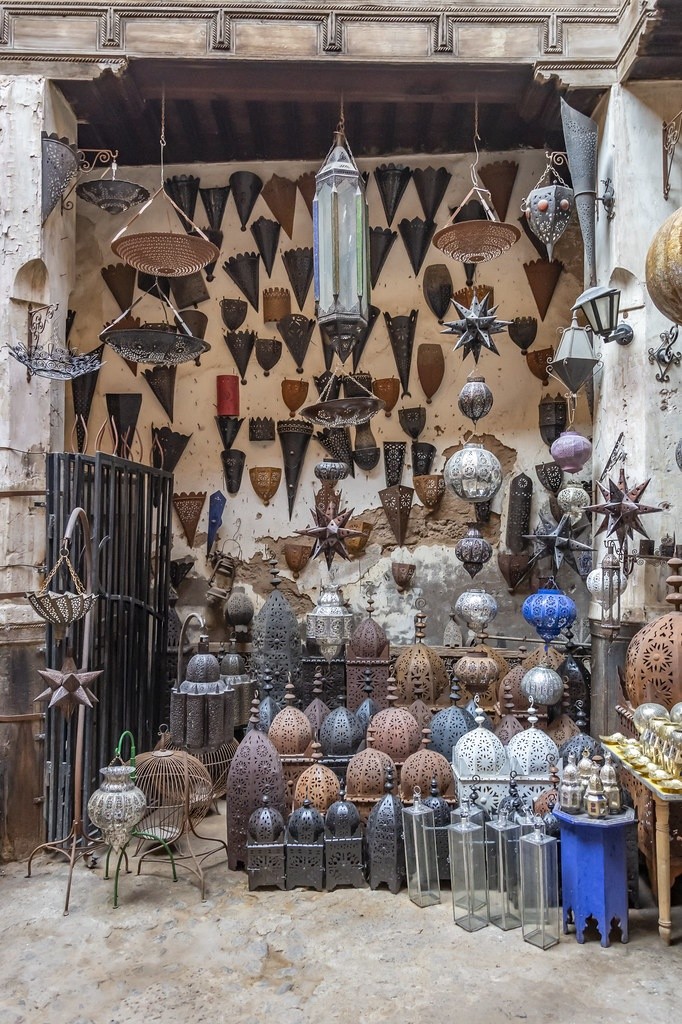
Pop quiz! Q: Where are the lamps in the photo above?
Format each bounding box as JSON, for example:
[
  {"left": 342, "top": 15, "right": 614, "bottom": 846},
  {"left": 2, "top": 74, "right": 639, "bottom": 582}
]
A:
[{"left": 571, "top": 285, "right": 632, "bottom": 346}]
